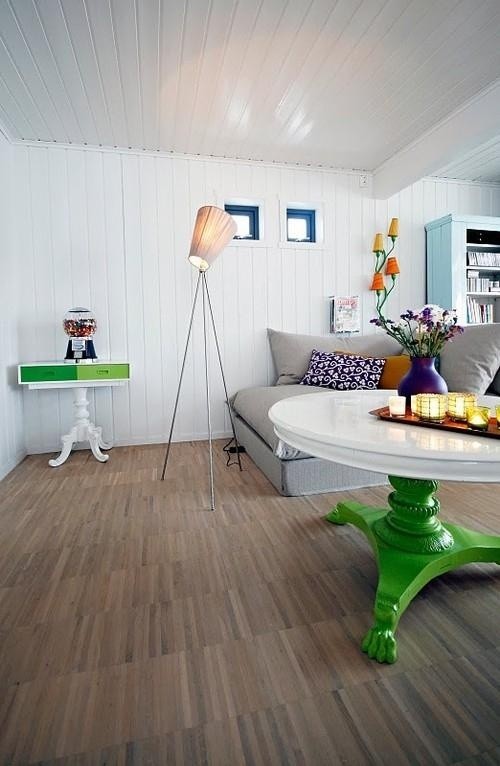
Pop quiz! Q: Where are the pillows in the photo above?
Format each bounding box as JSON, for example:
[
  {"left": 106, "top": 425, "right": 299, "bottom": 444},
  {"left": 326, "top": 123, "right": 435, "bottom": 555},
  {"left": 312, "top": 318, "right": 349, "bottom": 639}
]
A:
[{"left": 266, "top": 323, "right": 499, "bottom": 391}]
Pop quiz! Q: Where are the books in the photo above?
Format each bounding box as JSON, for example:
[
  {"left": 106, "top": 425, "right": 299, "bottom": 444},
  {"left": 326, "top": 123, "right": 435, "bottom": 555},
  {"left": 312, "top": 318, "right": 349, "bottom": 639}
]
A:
[{"left": 467, "top": 251, "right": 499, "bottom": 325}]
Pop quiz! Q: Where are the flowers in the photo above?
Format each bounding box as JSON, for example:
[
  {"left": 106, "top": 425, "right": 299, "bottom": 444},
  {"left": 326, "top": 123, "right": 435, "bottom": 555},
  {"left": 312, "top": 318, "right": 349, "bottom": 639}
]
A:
[{"left": 369, "top": 306, "right": 465, "bottom": 358}]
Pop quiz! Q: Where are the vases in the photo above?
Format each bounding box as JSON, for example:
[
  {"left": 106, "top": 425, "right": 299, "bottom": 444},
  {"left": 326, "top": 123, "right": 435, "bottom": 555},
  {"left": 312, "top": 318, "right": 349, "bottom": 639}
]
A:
[{"left": 397, "top": 356, "right": 448, "bottom": 405}]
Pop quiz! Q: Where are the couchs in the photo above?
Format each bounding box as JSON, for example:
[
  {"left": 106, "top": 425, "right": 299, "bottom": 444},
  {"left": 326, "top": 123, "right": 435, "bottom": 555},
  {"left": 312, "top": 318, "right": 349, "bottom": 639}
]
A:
[{"left": 227, "top": 368, "right": 500, "bottom": 497}]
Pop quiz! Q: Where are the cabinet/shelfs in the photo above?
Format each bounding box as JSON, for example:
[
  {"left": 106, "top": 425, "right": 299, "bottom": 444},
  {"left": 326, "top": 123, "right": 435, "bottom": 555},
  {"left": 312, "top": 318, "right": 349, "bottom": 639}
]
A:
[{"left": 424, "top": 213, "right": 500, "bottom": 326}]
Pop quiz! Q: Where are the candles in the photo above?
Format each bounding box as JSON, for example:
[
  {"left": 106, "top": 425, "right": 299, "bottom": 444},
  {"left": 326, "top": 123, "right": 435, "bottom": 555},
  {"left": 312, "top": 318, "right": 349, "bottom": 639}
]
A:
[{"left": 389, "top": 392, "right": 499, "bottom": 432}]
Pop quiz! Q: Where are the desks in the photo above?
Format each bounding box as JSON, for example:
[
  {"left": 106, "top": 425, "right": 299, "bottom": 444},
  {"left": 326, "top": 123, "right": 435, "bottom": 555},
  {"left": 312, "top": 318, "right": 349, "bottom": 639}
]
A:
[
  {"left": 267, "top": 388, "right": 499, "bottom": 664},
  {"left": 18, "top": 359, "right": 131, "bottom": 466}
]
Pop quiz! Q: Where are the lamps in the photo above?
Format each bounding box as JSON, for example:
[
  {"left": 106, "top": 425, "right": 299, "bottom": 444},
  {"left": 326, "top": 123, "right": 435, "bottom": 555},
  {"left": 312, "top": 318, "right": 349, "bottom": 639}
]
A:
[
  {"left": 160, "top": 205, "right": 243, "bottom": 511},
  {"left": 372, "top": 218, "right": 400, "bottom": 317}
]
